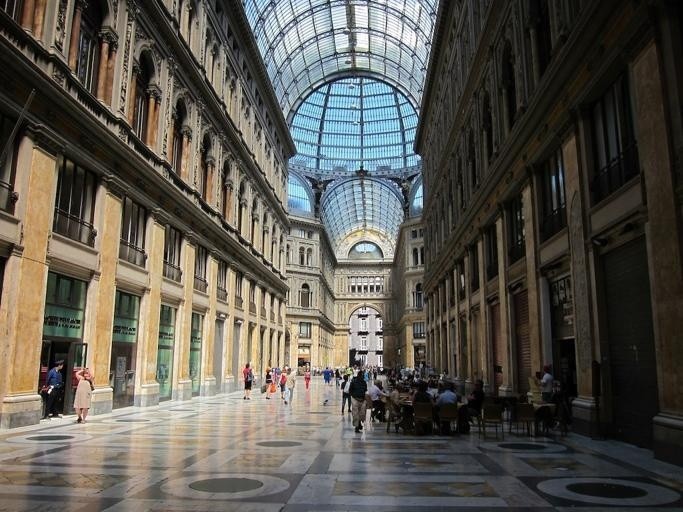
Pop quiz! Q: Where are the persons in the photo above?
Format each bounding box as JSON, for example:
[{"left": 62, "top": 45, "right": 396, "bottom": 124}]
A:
[
  {"left": 304, "top": 369, "right": 311, "bottom": 389},
  {"left": 242, "top": 363, "right": 253, "bottom": 400},
  {"left": 73, "top": 367, "right": 95, "bottom": 423},
  {"left": 338, "top": 364, "right": 482, "bottom": 438},
  {"left": 321, "top": 367, "right": 330, "bottom": 384},
  {"left": 45, "top": 359, "right": 64, "bottom": 417},
  {"left": 534, "top": 366, "right": 559, "bottom": 431},
  {"left": 334, "top": 369, "right": 341, "bottom": 386},
  {"left": 264, "top": 367, "right": 272, "bottom": 399},
  {"left": 283, "top": 368, "right": 295, "bottom": 405},
  {"left": 329, "top": 369, "right": 333, "bottom": 377},
  {"left": 278, "top": 368, "right": 286, "bottom": 399}
]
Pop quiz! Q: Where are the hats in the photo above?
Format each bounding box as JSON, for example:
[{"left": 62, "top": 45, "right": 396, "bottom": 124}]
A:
[{"left": 54, "top": 359, "right": 64, "bottom": 365}]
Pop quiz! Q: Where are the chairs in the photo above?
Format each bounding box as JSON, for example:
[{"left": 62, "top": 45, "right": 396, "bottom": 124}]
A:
[{"left": 364, "top": 373, "right": 540, "bottom": 441}]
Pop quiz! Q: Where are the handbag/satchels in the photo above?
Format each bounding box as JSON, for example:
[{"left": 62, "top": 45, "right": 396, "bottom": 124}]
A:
[
  {"left": 261, "top": 385, "right": 267, "bottom": 393},
  {"left": 271, "top": 384, "right": 276, "bottom": 393},
  {"left": 90, "top": 384, "right": 94, "bottom": 391},
  {"left": 247, "top": 372, "right": 254, "bottom": 382},
  {"left": 344, "top": 381, "right": 350, "bottom": 393},
  {"left": 279, "top": 376, "right": 286, "bottom": 386}
]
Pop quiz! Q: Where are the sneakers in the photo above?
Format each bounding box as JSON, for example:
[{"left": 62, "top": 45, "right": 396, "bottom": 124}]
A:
[
  {"left": 342, "top": 409, "right": 385, "bottom": 433},
  {"left": 244, "top": 396, "right": 292, "bottom": 406},
  {"left": 49, "top": 415, "right": 86, "bottom": 424}
]
